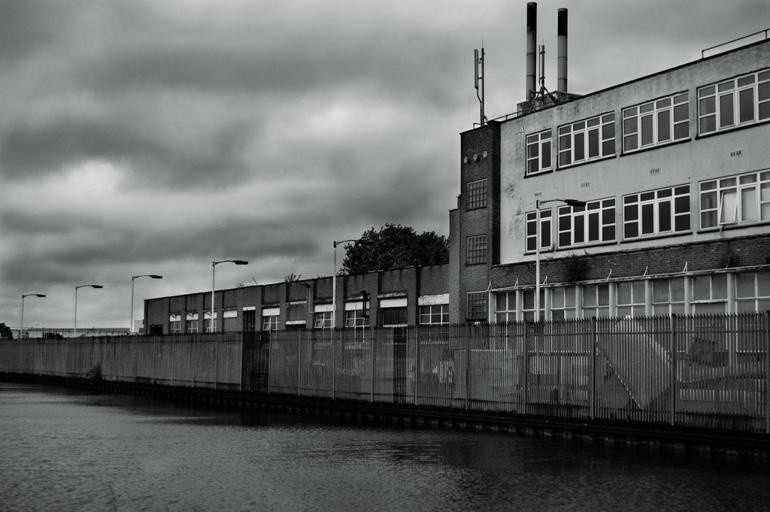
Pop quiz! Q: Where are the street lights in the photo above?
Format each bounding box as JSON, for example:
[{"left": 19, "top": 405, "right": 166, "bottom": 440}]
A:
[
  {"left": 130, "top": 274, "right": 162, "bottom": 331},
  {"left": 211, "top": 259, "right": 248, "bottom": 334},
  {"left": 74, "top": 284, "right": 103, "bottom": 334},
  {"left": 20, "top": 294, "right": 46, "bottom": 338},
  {"left": 333, "top": 240, "right": 375, "bottom": 327},
  {"left": 533, "top": 198, "right": 583, "bottom": 321}
]
